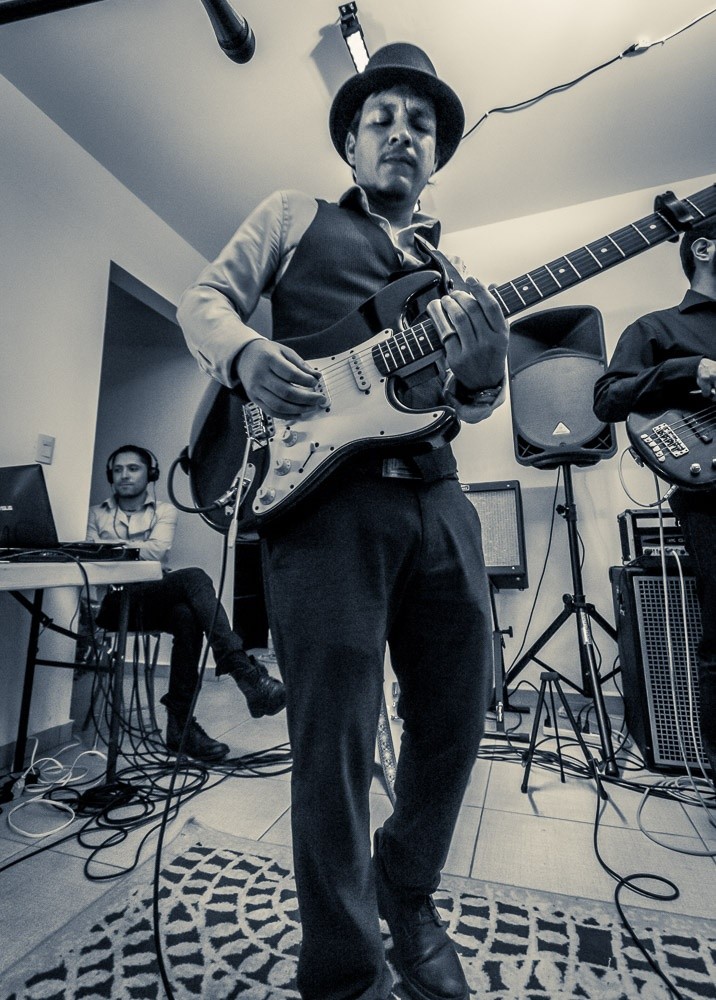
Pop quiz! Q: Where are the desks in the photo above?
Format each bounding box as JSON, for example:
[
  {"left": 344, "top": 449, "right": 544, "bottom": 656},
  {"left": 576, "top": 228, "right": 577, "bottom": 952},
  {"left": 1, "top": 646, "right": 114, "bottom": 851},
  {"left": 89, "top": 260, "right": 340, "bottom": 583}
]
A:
[{"left": 0, "top": 560, "right": 163, "bottom": 808}]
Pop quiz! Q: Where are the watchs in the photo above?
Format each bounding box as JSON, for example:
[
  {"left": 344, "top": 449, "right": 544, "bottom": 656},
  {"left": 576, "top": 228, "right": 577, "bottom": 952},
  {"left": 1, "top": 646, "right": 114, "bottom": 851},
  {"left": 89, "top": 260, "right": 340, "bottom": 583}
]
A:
[{"left": 456, "top": 374, "right": 504, "bottom": 404}]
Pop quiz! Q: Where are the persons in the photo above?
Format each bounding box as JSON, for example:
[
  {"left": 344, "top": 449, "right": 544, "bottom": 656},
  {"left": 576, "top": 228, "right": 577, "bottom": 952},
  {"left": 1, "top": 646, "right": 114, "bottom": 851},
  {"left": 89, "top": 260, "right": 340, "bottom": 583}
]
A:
[
  {"left": 591, "top": 218, "right": 716, "bottom": 786},
  {"left": 173, "top": 41, "right": 509, "bottom": 1000},
  {"left": 79, "top": 444, "right": 286, "bottom": 763}
]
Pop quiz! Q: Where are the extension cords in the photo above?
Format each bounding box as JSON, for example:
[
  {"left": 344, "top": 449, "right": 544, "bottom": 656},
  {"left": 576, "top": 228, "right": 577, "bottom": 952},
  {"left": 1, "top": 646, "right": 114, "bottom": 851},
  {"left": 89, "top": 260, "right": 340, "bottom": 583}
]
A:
[{"left": 0, "top": 774, "right": 38, "bottom": 802}]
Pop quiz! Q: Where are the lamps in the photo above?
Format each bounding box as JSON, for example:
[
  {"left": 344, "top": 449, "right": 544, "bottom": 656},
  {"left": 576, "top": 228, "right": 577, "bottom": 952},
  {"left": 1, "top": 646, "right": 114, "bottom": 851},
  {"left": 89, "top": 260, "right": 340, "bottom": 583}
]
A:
[{"left": 338, "top": 1, "right": 370, "bottom": 73}]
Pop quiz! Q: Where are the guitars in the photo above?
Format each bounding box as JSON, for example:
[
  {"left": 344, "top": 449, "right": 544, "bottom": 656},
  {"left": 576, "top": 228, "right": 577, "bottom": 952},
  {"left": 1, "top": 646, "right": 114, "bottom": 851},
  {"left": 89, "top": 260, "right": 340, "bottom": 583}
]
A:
[{"left": 188, "top": 183, "right": 716, "bottom": 544}]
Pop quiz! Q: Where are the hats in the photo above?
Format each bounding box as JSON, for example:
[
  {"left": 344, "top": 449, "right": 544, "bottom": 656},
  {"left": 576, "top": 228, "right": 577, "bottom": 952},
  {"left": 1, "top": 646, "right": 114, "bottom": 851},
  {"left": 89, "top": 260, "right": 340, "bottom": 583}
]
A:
[{"left": 329, "top": 42, "right": 465, "bottom": 174}]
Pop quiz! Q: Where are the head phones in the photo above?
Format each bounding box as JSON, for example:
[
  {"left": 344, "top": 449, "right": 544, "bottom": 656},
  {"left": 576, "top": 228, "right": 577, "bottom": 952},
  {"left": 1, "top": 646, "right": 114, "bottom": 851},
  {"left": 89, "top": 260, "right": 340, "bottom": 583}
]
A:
[{"left": 106, "top": 448, "right": 160, "bottom": 484}]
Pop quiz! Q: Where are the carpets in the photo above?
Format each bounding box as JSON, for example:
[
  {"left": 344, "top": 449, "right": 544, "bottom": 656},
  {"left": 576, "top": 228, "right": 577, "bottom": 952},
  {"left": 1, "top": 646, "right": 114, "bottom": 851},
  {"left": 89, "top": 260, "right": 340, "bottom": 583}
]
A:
[{"left": 0, "top": 816, "right": 716, "bottom": 1000}]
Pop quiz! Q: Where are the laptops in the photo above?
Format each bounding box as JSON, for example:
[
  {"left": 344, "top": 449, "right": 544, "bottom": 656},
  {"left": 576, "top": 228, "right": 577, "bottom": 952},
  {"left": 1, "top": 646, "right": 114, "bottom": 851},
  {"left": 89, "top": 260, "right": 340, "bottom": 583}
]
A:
[{"left": 0, "top": 465, "right": 127, "bottom": 550}]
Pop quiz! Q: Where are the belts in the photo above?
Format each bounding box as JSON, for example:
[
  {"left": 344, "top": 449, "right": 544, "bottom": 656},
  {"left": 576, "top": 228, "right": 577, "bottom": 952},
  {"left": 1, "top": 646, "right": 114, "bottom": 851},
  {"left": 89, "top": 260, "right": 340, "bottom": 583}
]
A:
[{"left": 334, "top": 450, "right": 459, "bottom": 480}]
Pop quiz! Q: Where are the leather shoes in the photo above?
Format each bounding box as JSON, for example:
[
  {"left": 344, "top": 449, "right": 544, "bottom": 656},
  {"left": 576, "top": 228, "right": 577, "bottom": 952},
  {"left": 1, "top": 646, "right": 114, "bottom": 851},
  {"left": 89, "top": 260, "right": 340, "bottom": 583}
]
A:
[
  {"left": 372, "top": 858, "right": 470, "bottom": 1000},
  {"left": 166, "top": 718, "right": 229, "bottom": 761},
  {"left": 235, "top": 665, "right": 291, "bottom": 719}
]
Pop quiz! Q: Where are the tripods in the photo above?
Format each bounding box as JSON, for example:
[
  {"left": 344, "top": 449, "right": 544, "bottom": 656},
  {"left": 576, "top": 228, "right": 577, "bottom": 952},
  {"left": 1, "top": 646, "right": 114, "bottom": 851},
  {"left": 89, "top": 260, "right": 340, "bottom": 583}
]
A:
[{"left": 507, "top": 464, "right": 620, "bottom": 799}]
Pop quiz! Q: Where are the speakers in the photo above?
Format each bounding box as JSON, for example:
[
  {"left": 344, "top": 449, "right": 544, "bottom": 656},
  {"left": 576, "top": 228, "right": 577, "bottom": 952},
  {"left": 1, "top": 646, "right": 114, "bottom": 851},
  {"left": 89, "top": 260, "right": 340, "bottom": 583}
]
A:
[
  {"left": 459, "top": 481, "right": 528, "bottom": 590},
  {"left": 506, "top": 304, "right": 618, "bottom": 470},
  {"left": 608, "top": 557, "right": 713, "bottom": 779}
]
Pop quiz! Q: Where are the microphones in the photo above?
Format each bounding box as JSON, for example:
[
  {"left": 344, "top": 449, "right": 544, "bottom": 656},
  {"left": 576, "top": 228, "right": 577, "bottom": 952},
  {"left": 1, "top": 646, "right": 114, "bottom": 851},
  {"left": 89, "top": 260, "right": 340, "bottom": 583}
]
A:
[{"left": 201, "top": 0, "right": 256, "bottom": 65}]
